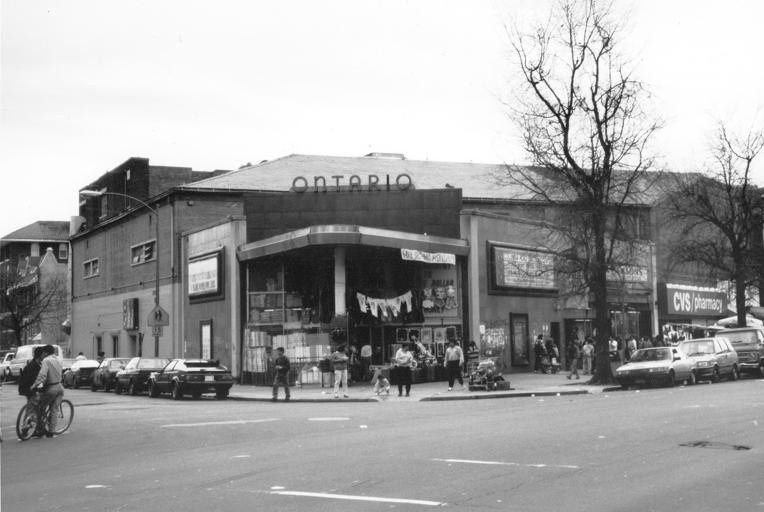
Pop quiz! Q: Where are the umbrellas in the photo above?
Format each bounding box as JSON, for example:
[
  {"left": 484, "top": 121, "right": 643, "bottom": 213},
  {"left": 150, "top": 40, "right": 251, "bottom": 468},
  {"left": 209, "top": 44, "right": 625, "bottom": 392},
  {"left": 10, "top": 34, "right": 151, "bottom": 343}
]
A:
[{"left": 671, "top": 313, "right": 763, "bottom": 340}]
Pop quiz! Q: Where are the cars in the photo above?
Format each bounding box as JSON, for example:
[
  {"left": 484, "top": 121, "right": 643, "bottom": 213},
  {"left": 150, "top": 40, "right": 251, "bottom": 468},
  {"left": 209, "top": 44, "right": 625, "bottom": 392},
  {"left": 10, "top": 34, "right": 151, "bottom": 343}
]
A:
[
  {"left": 679, "top": 335, "right": 740, "bottom": 383},
  {"left": 0, "top": 344, "right": 235, "bottom": 399},
  {"left": 618, "top": 346, "right": 698, "bottom": 390}
]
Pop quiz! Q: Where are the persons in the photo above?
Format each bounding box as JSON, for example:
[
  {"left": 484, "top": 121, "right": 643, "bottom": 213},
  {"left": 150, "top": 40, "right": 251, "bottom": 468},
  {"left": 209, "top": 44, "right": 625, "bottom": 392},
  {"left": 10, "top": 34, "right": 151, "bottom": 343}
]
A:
[
  {"left": 374, "top": 374, "right": 390, "bottom": 396},
  {"left": 395, "top": 343, "right": 413, "bottom": 397},
  {"left": 533, "top": 330, "right": 686, "bottom": 380},
  {"left": 96, "top": 351, "right": 106, "bottom": 363},
  {"left": 30, "top": 345, "right": 64, "bottom": 438},
  {"left": 17, "top": 347, "right": 46, "bottom": 437},
  {"left": 271, "top": 346, "right": 291, "bottom": 402},
  {"left": 443, "top": 339, "right": 466, "bottom": 392},
  {"left": 75, "top": 352, "right": 87, "bottom": 361},
  {"left": 331, "top": 343, "right": 371, "bottom": 398}
]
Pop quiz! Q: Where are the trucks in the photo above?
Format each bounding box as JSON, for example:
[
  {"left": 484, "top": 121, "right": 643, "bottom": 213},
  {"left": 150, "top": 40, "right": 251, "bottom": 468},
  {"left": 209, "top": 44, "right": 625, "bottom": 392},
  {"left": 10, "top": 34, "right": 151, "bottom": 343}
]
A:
[{"left": 715, "top": 327, "right": 764, "bottom": 376}]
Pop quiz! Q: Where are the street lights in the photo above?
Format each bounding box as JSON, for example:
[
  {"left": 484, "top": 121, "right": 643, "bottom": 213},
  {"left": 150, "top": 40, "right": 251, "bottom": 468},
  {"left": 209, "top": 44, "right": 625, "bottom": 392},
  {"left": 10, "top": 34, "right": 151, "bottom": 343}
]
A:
[{"left": 78, "top": 189, "right": 160, "bottom": 357}]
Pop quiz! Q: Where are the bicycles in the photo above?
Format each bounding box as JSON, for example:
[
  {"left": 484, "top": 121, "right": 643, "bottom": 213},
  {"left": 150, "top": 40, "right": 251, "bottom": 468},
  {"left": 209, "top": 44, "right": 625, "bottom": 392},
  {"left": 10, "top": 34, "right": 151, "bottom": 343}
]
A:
[{"left": 15, "top": 387, "right": 76, "bottom": 441}]
